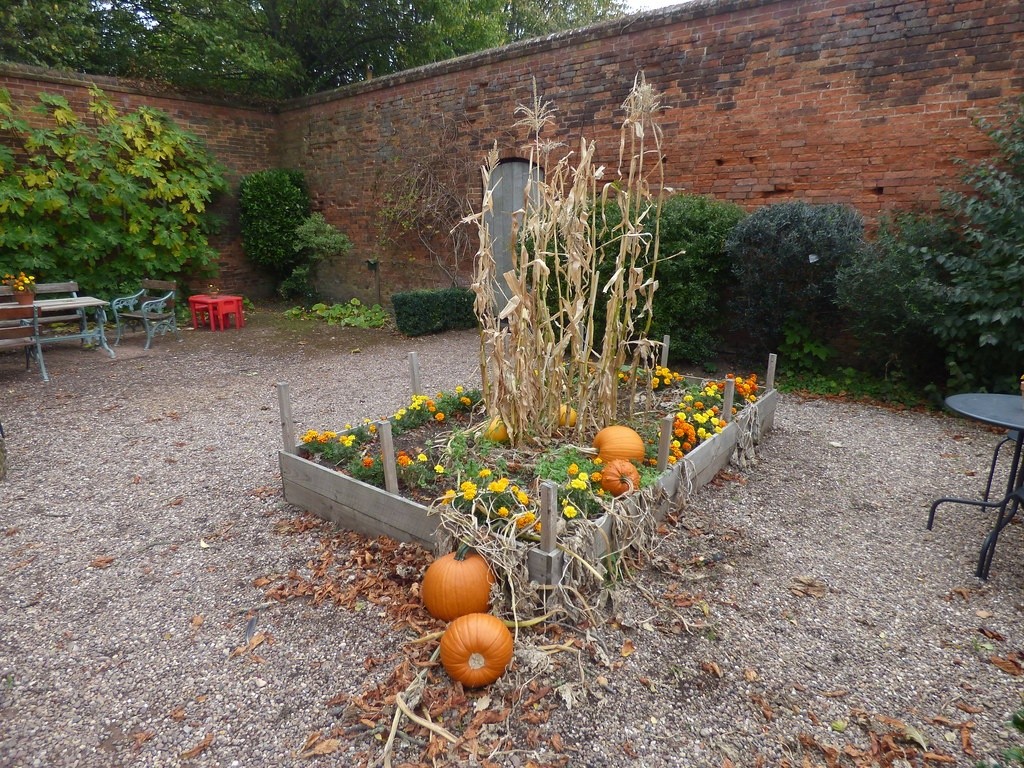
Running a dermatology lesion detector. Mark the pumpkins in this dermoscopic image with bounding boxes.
[423,539,496,622]
[441,613,514,688]
[559,405,577,426]
[576,426,645,464]
[601,460,639,498]
[482,415,508,442]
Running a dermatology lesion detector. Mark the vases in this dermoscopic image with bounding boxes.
[15,291,34,305]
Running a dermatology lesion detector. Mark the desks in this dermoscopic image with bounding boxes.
[188,294,243,331]
[0,297,116,359]
[928,392,1024,579]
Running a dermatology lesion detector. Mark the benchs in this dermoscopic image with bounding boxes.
[0,305,49,381]
[0,282,88,345]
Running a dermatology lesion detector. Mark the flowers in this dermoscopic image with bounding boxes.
[2,271,35,292]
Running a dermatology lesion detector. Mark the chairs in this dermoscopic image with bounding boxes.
[211,298,240,331]
[194,295,209,326]
[112,278,177,351]
